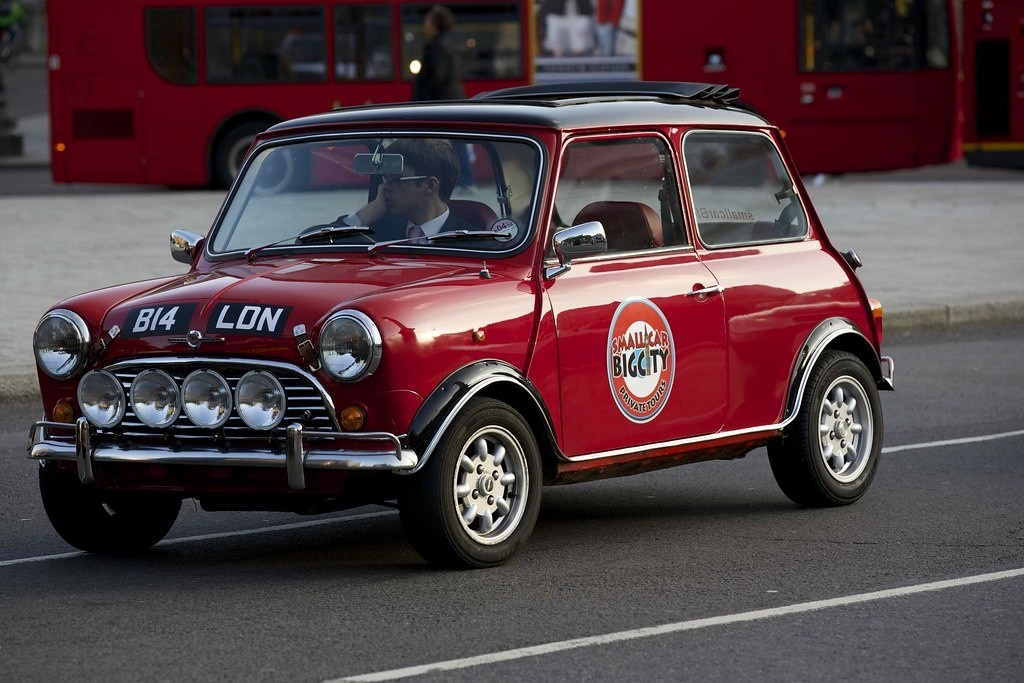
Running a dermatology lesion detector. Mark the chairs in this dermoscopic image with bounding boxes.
[572,200,663,250]
[450,199,498,229]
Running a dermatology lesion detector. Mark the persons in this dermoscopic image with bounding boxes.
[408,7,479,198]
[340,137,500,245]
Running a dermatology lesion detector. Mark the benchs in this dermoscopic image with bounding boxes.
[670,221,775,245]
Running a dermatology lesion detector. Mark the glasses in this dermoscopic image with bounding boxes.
[382,175,439,185]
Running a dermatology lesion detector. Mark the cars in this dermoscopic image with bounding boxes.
[24,80,896,570]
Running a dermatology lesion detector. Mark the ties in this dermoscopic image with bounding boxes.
[409,226,423,243]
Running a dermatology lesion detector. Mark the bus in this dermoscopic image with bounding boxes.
[47,0,963,196]
